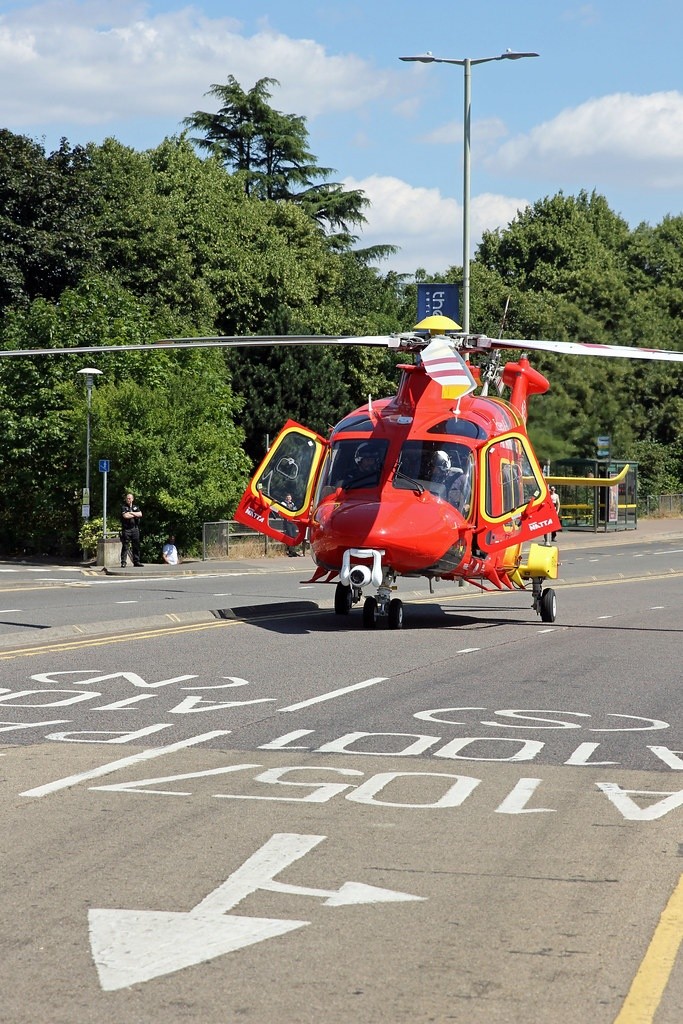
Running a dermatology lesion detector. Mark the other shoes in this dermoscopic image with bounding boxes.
[134,561,144,567]
[121,561,127,568]
[289,550,297,557]
[552,536,558,542]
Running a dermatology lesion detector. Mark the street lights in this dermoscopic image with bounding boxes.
[398,47,541,362]
[76,367,103,560]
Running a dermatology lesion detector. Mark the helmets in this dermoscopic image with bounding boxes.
[354,441,384,466]
[430,449,451,471]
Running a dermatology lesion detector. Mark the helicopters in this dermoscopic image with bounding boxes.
[0,307,683,631]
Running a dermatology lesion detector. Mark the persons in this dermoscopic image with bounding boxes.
[278,492,299,557]
[426,450,470,514]
[341,442,384,488]
[119,493,144,568]
[544,486,560,542]
